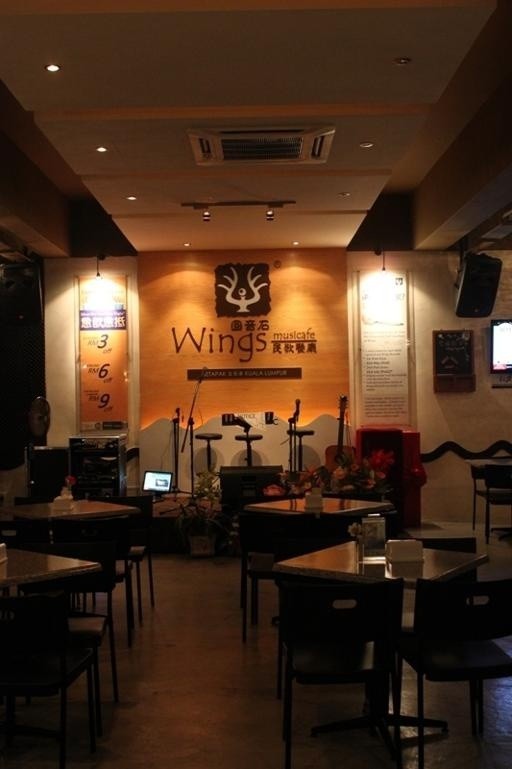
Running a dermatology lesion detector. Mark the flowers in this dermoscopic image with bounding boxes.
[262,447,396,508]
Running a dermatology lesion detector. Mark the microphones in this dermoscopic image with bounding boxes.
[196,364,208,386]
[295,399,301,420]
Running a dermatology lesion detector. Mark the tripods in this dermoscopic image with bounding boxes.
[159,382,221,515]
[153,408,200,502]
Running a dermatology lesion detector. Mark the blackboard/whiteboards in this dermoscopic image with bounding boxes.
[433,330,476,393]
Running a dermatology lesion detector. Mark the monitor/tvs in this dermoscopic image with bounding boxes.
[142,470,174,503]
[491,318,512,374]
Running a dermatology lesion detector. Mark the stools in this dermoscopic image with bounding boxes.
[194,429,314,499]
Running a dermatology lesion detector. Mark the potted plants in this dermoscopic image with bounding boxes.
[156,467,237,556]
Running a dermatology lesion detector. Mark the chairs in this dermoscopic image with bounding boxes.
[471,464,511,544]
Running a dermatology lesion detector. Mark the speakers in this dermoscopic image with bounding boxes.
[220,465,282,503]
[0,264,44,327]
[24,433,69,499]
[454,250,503,319]
[64,434,126,502]
